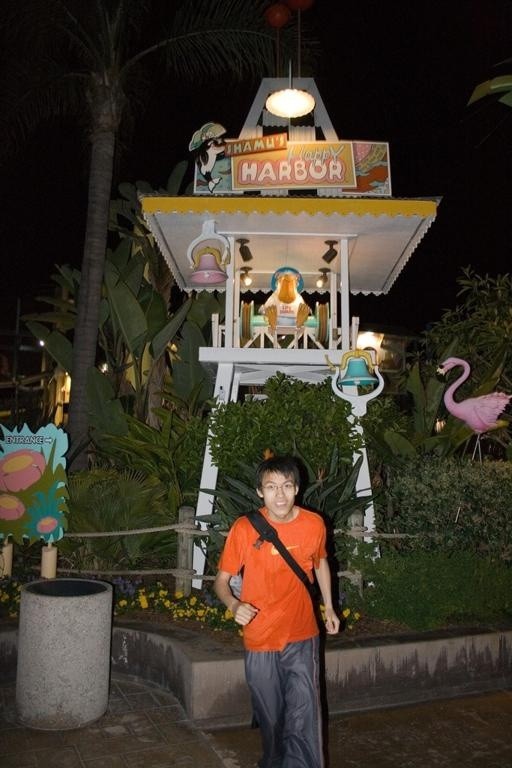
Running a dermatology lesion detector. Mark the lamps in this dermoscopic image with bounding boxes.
[236,238,253,261]
[322,239,339,263]
[240,266,254,287]
[265,54,316,120]
[316,268,331,288]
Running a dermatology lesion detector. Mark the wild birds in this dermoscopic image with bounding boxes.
[437,358,512,435]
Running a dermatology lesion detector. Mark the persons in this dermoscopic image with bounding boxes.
[213,456,343,767]
